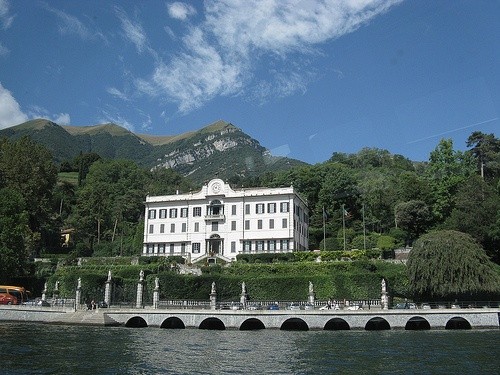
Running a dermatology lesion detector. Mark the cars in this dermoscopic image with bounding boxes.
[392,302,500,309]
[220,302,362,310]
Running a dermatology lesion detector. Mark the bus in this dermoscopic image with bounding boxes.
[0,285,30,305]
